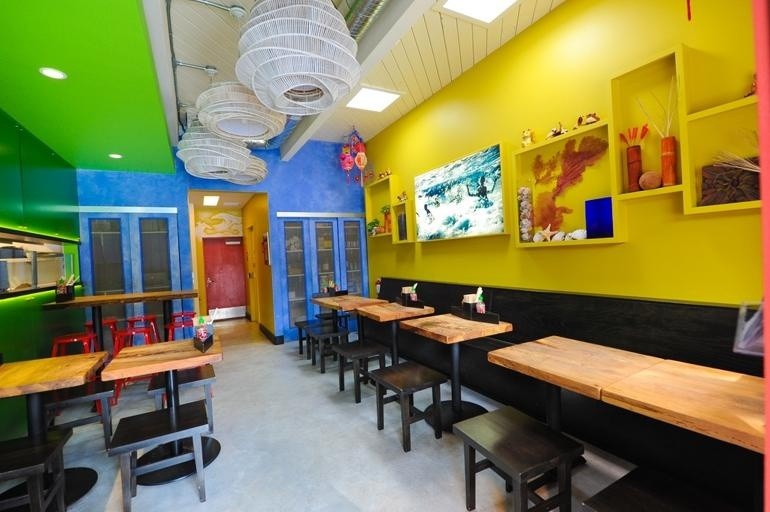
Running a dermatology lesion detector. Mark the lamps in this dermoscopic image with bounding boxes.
[176,100,252,179]
[217,156,269,186]
[186,0,361,117]
[173,57,287,143]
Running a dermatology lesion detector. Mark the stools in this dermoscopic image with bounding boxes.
[47,332,98,411]
[0,427,74,511]
[584,466,764,512]
[43,380,116,440]
[306,325,348,375]
[452,405,585,512]
[316,311,351,345]
[113,326,157,406]
[84,318,115,350]
[107,399,211,512]
[128,315,162,345]
[368,359,448,453]
[332,338,391,403]
[166,320,205,340]
[147,364,216,430]
[294,320,317,354]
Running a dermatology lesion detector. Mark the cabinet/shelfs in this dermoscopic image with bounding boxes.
[79,212,182,350]
[339,217,371,337]
[281,216,343,343]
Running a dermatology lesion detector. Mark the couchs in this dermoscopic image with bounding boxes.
[366,278,764,510]
